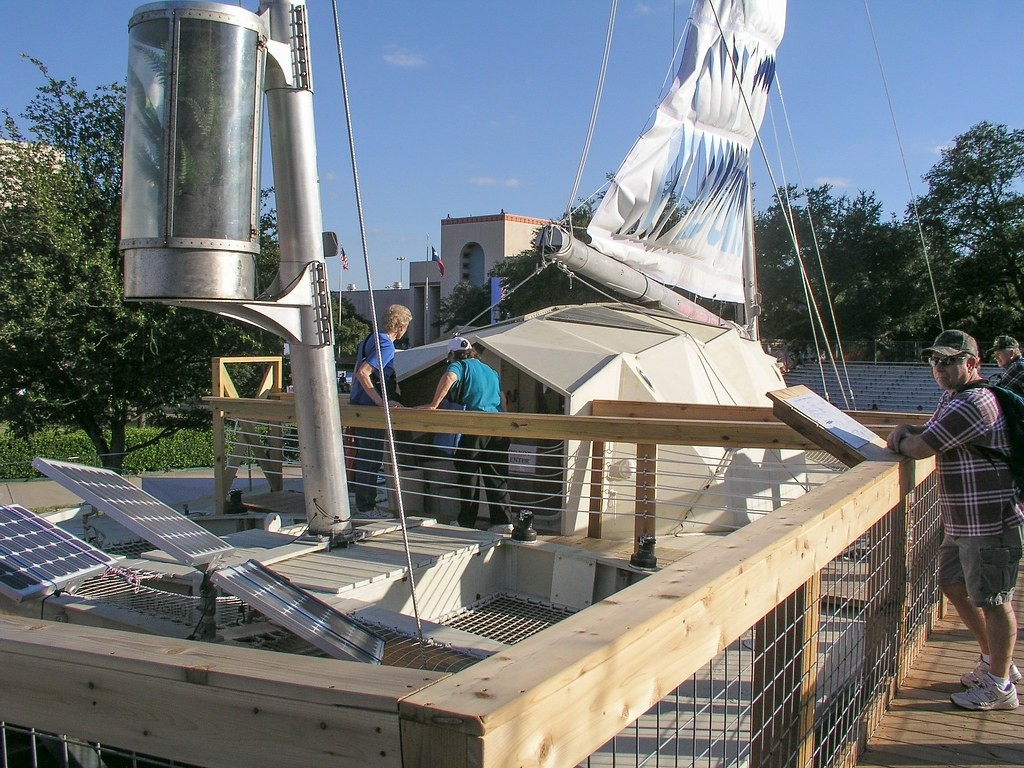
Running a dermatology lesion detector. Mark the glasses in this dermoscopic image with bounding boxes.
[928,353,969,366]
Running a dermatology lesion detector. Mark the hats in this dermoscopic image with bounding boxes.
[447,336,472,358]
[986,335,1019,354]
[921,330,978,357]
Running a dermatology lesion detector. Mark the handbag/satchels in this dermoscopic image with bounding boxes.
[432,358,466,454]
[370,372,403,403]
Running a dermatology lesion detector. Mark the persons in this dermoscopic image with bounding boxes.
[985,333,1024,398]
[884,328,1024,713]
[413,332,518,532]
[349,301,413,517]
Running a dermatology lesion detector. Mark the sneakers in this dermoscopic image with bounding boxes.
[950,672,1019,710]
[959,654,1022,688]
[450,520,460,527]
[375,493,387,502]
[487,524,515,534]
[356,507,395,518]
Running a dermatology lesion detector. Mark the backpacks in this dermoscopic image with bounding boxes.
[957,374,1024,499]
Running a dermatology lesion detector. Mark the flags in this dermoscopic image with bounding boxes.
[340,246,349,271]
[431,246,445,277]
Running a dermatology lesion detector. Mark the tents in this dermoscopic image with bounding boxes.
[385,299,817,540]
